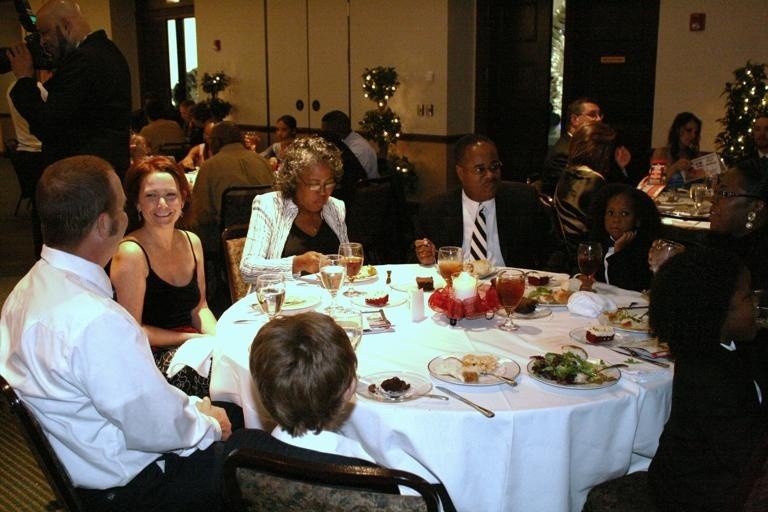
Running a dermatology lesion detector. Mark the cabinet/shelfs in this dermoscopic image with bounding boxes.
[264,0,350,137]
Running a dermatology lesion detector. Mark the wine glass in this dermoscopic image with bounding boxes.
[496,269,526,331]
[690,184,708,213]
[318,254,346,311]
[753,288,768,329]
[338,242,364,296]
[639,239,684,302]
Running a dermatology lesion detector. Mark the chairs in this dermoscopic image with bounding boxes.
[526,177,574,273]
[220,223,253,303]
[221,448,440,512]
[0,377,84,512]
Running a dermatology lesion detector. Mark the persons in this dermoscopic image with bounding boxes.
[109,156,218,400]
[239,133,354,294]
[539,97,768,309]
[413,135,554,270]
[5,0,132,185]
[129,82,381,228]
[1,155,244,512]
[250,311,379,465]
[649,254,768,512]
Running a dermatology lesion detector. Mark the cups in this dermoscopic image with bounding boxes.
[438,247,463,287]
[246,131,255,146]
[328,308,363,353]
[256,274,286,322]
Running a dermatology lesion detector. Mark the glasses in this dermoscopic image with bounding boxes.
[298,176,337,191]
[470,161,503,175]
[576,113,605,121]
[716,191,759,199]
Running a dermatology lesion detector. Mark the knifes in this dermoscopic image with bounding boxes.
[386,270,392,284]
[610,348,669,368]
[435,386,495,418]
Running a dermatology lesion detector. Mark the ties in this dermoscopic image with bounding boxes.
[468,203,487,263]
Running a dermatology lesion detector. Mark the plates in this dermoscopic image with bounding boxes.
[569,327,633,347]
[495,305,551,320]
[527,356,622,390]
[316,264,378,283]
[525,287,570,307]
[428,353,521,386]
[257,287,321,311]
[356,371,433,403]
[598,308,648,333]
